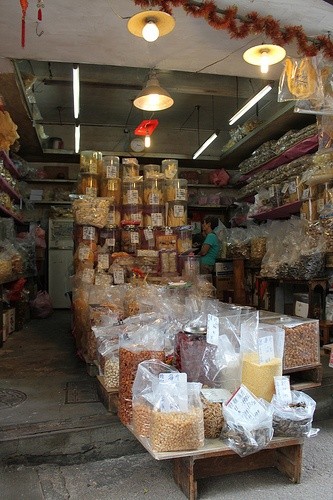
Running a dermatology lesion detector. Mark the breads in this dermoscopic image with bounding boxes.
[161,253,177,272]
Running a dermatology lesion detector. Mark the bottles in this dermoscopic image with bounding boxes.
[48,137,64,149]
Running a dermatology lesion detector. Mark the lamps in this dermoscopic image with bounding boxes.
[242,38,289,73]
[131,64,175,112]
[227,74,276,127]
[70,62,82,126]
[75,122,83,155]
[192,95,221,159]
[125,6,178,43]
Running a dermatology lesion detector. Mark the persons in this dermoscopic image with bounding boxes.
[198,215,220,274]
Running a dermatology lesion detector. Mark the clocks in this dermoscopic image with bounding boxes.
[127,136,147,153]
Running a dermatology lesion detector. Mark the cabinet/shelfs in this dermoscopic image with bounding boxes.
[178,73,333,311]
[0,65,79,316]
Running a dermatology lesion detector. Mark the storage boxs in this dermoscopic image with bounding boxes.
[252,310,321,370]
